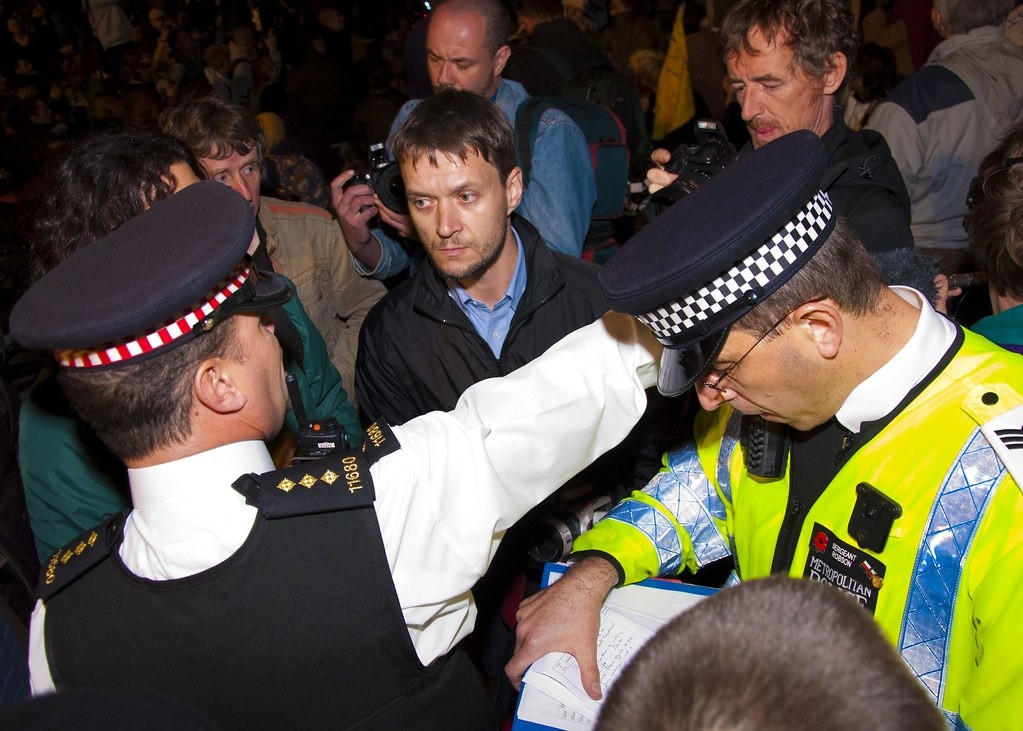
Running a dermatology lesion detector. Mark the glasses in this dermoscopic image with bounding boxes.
[695,294,828,394]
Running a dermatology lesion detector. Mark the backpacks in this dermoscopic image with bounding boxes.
[514,97,629,239]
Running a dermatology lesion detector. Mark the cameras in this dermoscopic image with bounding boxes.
[353,143,409,217]
[524,495,612,561]
[643,141,728,219]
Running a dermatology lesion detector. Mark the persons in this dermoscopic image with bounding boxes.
[352,87,674,724]
[0,0,1023,722]
[10,179,661,731]
[502,128,1023,731]
[644,1,910,269]
[592,571,951,730]
[18,127,365,567]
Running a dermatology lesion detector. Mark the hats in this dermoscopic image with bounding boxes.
[597,129,836,397]
[9,179,293,371]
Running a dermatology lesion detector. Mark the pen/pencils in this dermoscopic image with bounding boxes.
[947,272,988,288]
